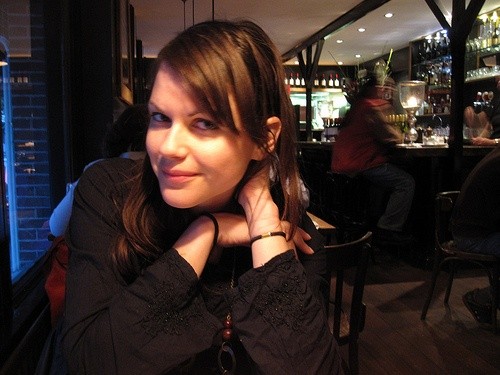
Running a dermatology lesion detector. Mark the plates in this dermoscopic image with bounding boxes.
[421,144,446,147]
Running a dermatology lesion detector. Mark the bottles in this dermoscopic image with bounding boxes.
[321,74,326,88]
[415,41,432,63]
[329,73,333,88]
[289,73,294,87]
[314,73,319,87]
[417,126,450,137]
[431,35,450,59]
[284,73,288,84]
[322,118,343,138]
[335,74,340,87]
[419,65,451,85]
[481,20,500,48]
[301,77,305,87]
[295,73,300,87]
[425,96,436,114]
[386,114,408,133]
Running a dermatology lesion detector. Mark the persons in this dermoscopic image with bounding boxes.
[49,104,149,237]
[330,74,415,236]
[463,76,500,145]
[450,145,500,329]
[53,20,348,374]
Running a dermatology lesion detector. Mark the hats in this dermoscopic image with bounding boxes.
[363,74,398,93]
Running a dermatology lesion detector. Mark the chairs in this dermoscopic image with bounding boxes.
[318,231,377,375]
[421,147,500,337]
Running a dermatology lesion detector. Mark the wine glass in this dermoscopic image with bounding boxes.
[409,134,418,146]
[476,92,493,102]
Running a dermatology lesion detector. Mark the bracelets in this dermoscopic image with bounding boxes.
[249,231,286,245]
[200,211,219,245]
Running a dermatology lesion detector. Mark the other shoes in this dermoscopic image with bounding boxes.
[373,227,417,246]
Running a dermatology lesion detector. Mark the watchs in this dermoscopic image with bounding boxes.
[494,138,499,145]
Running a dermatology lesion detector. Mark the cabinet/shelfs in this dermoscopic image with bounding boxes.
[406,7,500,137]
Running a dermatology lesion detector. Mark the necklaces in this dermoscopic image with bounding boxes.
[223,252,239,343]
